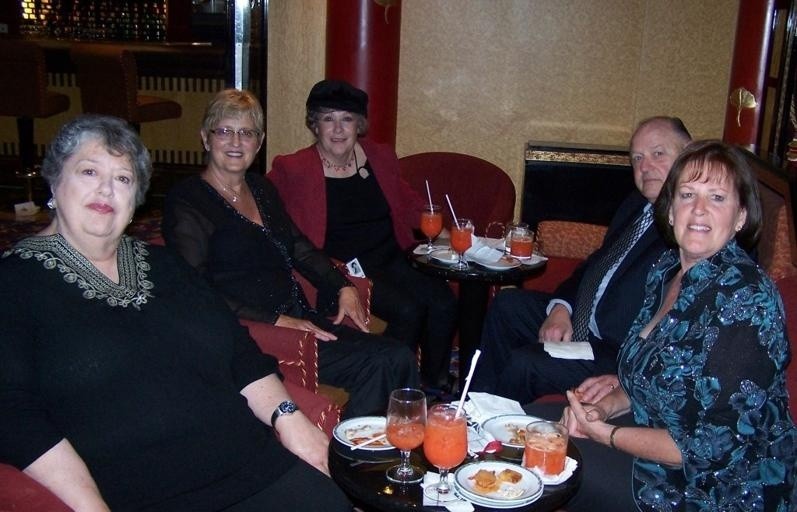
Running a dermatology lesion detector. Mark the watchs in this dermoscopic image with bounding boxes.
[266,398,301,433]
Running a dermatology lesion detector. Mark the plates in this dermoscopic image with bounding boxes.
[332,416,398,451]
[453,459,545,510]
[429,250,457,264]
[474,254,522,271]
[481,414,548,449]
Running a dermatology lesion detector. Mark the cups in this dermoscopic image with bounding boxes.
[525,420,570,475]
[507,222,535,259]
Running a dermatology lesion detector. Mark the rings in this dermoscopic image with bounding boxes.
[363,320,369,325]
[608,383,615,389]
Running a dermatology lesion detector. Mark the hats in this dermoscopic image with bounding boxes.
[306,80,368,119]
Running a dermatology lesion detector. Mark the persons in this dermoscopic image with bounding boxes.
[157,88,426,419]
[347,261,361,275]
[520,138,797,512]
[0,113,357,512]
[263,77,464,395]
[464,114,695,404]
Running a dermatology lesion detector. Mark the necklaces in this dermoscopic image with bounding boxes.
[316,142,360,171]
[215,175,249,204]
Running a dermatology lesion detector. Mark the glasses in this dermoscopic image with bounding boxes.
[211,127,260,140]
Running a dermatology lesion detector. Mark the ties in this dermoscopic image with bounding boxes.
[571,204,654,341]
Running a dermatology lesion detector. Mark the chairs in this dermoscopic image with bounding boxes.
[397,150,516,237]
[1,34,182,191]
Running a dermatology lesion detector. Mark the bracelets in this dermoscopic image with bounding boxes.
[611,422,621,448]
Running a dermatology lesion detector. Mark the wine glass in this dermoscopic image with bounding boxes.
[423,404,469,502]
[384,388,428,484]
[421,205,444,251]
[451,220,472,270]
